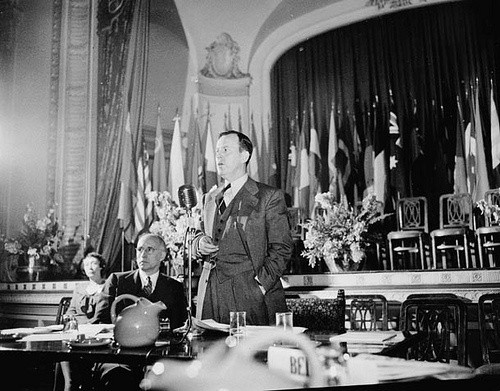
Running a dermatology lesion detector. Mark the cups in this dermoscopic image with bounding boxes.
[229,311,246,339]
[276,312,293,330]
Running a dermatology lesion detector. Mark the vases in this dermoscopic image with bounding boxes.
[18,252,56,282]
[323,255,363,271]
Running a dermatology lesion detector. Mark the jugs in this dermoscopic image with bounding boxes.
[110,294,167,347]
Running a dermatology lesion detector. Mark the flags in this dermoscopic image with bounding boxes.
[117,107,279,245]
[282,79,500,230]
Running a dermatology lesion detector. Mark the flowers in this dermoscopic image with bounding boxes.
[2,200,70,261]
[476,199,500,225]
[149,188,208,275]
[297,192,393,265]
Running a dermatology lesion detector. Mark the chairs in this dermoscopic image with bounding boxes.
[384,189,500,271]
[289,290,500,367]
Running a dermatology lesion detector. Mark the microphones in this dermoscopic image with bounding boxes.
[178,184,197,211]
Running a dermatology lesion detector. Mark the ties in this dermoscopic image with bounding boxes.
[143,276,152,298]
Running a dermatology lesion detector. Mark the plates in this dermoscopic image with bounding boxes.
[69,337,112,347]
[276,341,322,348]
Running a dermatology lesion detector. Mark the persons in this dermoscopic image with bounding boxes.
[54,252,107,391]
[187,130,295,326]
[90,234,187,391]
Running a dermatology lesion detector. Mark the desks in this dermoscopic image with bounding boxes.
[0,331,193,391]
[273,362,480,390]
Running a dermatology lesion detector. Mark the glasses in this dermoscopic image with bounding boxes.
[135,247,163,255]
